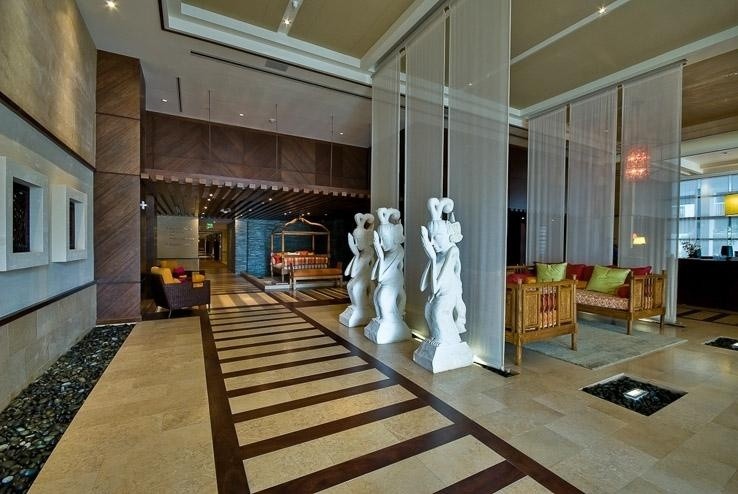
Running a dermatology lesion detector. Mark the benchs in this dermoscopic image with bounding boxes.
[506,262,666,364]
[289,262,343,290]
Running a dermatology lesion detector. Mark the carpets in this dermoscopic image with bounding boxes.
[508,317,686,370]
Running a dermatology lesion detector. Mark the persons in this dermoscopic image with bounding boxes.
[416,196,469,342]
[366,205,409,319]
[341,211,377,309]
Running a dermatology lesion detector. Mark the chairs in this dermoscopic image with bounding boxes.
[144,261,210,319]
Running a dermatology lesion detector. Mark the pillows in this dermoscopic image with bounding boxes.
[271,255,281,264]
[506,261,653,305]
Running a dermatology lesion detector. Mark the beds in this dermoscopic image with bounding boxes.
[270,251,328,283]
